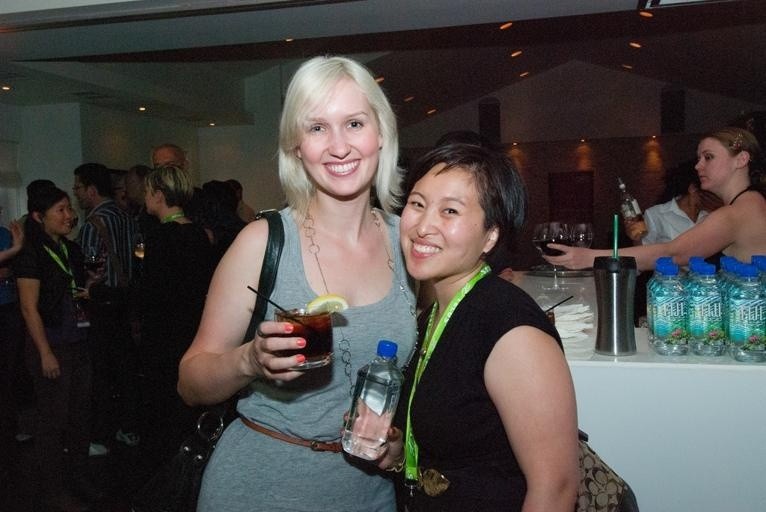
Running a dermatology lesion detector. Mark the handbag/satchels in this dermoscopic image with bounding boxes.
[573,429,639,511]
[128,207,285,511]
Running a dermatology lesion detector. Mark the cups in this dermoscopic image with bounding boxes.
[572,223,592,247]
[594,256,638,356]
[274,303,334,372]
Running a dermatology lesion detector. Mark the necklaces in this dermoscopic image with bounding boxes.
[300,207,418,400]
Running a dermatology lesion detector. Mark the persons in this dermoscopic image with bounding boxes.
[176,56,424,512]
[624,170,721,246]
[2,142,254,512]
[340,143,579,511]
[541,128,766,272]
[431,130,514,281]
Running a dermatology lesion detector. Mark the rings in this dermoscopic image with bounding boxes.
[572,265,576,269]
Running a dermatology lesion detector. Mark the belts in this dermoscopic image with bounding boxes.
[239,413,342,454]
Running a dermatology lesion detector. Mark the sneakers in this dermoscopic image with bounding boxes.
[114,429,139,448]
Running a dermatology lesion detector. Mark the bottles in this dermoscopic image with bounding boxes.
[646,255,766,363]
[616,177,649,239]
[341,339,405,462]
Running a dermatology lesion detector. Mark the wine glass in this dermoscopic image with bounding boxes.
[533,222,571,289]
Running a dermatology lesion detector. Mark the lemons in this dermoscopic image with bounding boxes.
[306,293,349,316]
